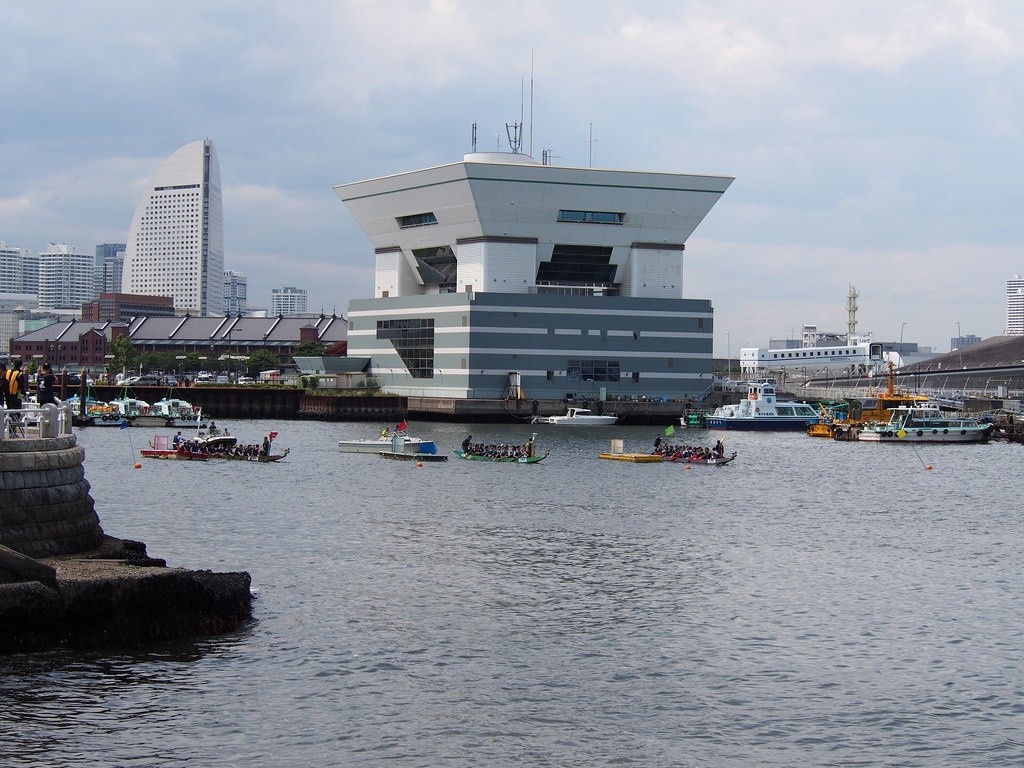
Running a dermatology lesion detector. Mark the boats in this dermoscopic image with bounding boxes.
[20,369,291,463]
[649,437,738,466]
[806,361,993,444]
[535,406,619,426]
[337,429,438,457]
[450,445,551,464]
[702,382,822,433]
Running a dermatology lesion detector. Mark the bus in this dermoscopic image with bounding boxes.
[757,377,778,390]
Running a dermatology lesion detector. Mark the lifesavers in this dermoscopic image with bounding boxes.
[187,416,191,420]
[686,403,691,408]
[193,416,197,420]
[102,416,107,421]
[917,430,923,436]
[181,416,186,420]
[597,400,604,412]
[837,428,842,436]
[713,403,718,408]
[933,429,937,434]
[882,433,886,437]
[114,415,119,420]
[109,415,114,420]
[584,401,588,407]
[887,431,893,437]
[756,407,760,412]
[961,430,967,435]
[895,430,908,437]
[532,400,539,411]
[132,416,135,421]
[943,429,948,434]
[200,417,203,420]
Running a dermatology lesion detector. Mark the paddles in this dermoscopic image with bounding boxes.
[483,451,527,462]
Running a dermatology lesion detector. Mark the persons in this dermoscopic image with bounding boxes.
[156,377,169,386]
[653,434,724,459]
[0,363,11,408]
[173,422,271,458]
[462,435,532,458]
[23,367,29,392]
[35,362,54,406]
[382,425,399,437]
[6,359,26,438]
[185,378,190,387]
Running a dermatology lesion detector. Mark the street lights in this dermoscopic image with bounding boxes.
[725,331,731,379]
[954,322,962,368]
[896,322,907,375]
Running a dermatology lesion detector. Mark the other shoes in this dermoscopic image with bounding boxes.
[10,433,19,438]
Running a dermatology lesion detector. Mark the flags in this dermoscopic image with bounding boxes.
[121,420,127,429]
[400,421,408,431]
[665,425,675,435]
[897,430,906,438]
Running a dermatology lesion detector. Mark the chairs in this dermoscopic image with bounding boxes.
[6,409,27,439]
